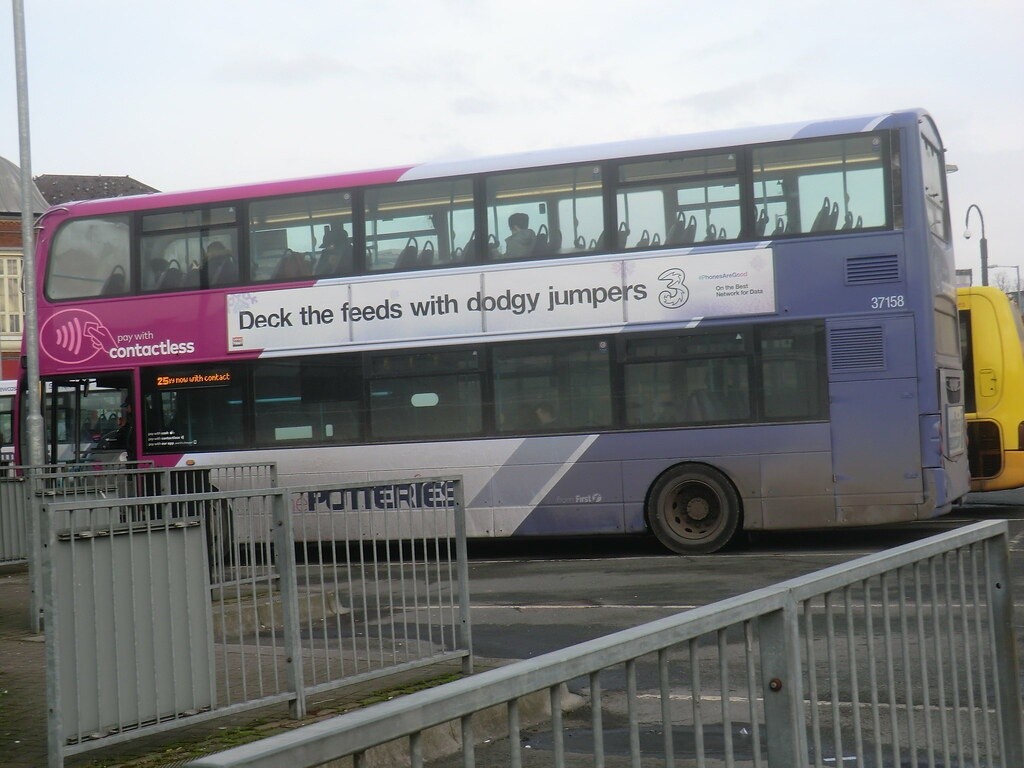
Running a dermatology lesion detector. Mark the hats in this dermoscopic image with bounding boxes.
[120,396,132,407]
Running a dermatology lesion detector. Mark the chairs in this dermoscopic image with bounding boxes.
[574,230,604,250]
[270,245,372,280]
[736,205,785,237]
[533,224,562,254]
[98,254,258,297]
[393,231,499,270]
[635,230,649,248]
[651,233,661,246]
[618,221,628,249]
[663,210,727,244]
[810,197,863,232]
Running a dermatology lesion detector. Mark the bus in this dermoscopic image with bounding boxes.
[956,285,1024,493]
[0,380,131,479]
[13,110,971,556]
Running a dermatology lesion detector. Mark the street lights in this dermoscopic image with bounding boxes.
[963,205,988,285]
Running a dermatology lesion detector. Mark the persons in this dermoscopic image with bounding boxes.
[492,213,535,259]
[191,241,231,288]
[535,401,559,428]
[116,395,149,463]
[327,231,352,271]
